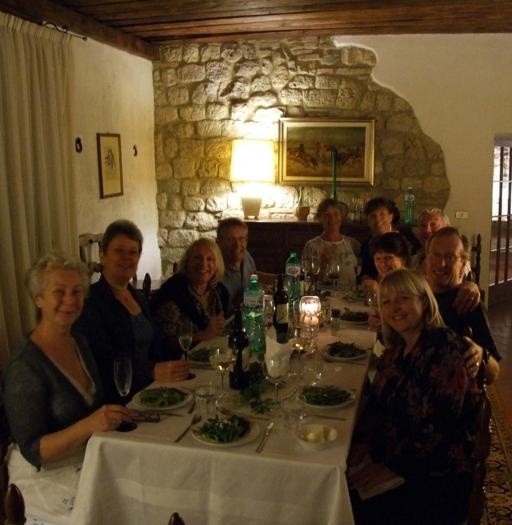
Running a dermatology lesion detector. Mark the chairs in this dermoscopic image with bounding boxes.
[78,232,105,275]
[470,233,485,307]
[0,442,25,525]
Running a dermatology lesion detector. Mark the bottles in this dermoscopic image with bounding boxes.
[293,186,310,221]
[348,195,370,223]
[403,186,416,224]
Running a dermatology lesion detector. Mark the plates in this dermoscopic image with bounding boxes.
[131,317,377,448]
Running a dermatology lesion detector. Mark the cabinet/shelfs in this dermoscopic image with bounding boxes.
[246,223,422,279]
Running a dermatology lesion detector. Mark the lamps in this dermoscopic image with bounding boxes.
[297,294,322,357]
[229,138,274,219]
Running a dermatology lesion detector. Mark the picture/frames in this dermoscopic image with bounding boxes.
[277,118,377,186]
[98,133,124,198]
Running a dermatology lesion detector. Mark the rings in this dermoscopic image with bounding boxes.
[175,378,178,382]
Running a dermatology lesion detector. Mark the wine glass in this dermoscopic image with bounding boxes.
[114,256,378,433]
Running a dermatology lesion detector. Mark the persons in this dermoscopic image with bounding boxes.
[302,196,502,384]
[344,270,493,525]
[149,238,240,343]
[215,218,256,304]
[71,219,191,406]
[2,250,133,525]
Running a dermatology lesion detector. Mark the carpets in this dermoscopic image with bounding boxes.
[481,381,511,525]
